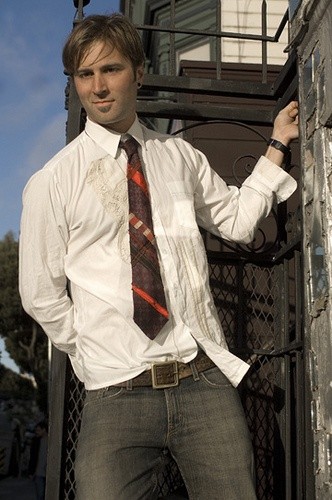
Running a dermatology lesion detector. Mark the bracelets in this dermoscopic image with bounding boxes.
[266,138,289,154]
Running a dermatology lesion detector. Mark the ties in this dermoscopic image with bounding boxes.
[119,137,170,341]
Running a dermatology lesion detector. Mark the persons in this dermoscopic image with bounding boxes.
[19,12,299,500]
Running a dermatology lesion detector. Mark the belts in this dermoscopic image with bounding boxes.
[110,349,215,390]
